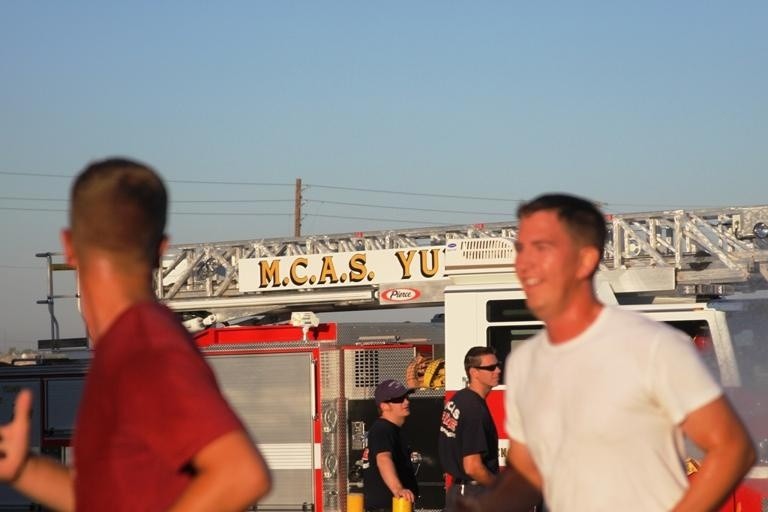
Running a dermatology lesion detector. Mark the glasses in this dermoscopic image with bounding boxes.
[473,360,502,372]
[386,393,410,403]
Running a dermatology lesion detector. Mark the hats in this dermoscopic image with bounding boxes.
[375,380,416,405]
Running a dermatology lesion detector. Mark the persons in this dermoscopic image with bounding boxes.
[360,376,419,512]
[505,194,758,510]
[434,347,503,511]
[1,155,274,511]
